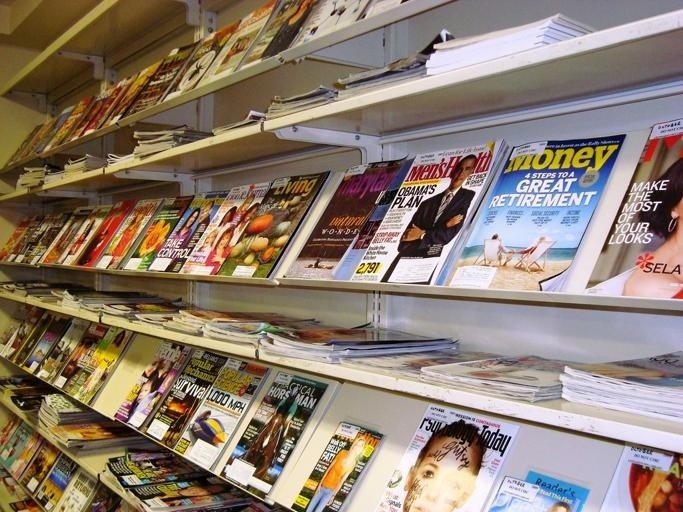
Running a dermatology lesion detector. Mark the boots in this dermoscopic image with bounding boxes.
[275,434,286,453]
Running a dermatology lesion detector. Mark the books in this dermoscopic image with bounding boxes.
[1,1,683,511]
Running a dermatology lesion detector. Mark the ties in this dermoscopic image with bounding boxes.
[434,193,453,224]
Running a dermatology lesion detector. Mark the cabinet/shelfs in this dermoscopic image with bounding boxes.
[0,0,683,512]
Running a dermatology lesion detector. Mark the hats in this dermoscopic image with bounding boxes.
[288,387,299,398]
